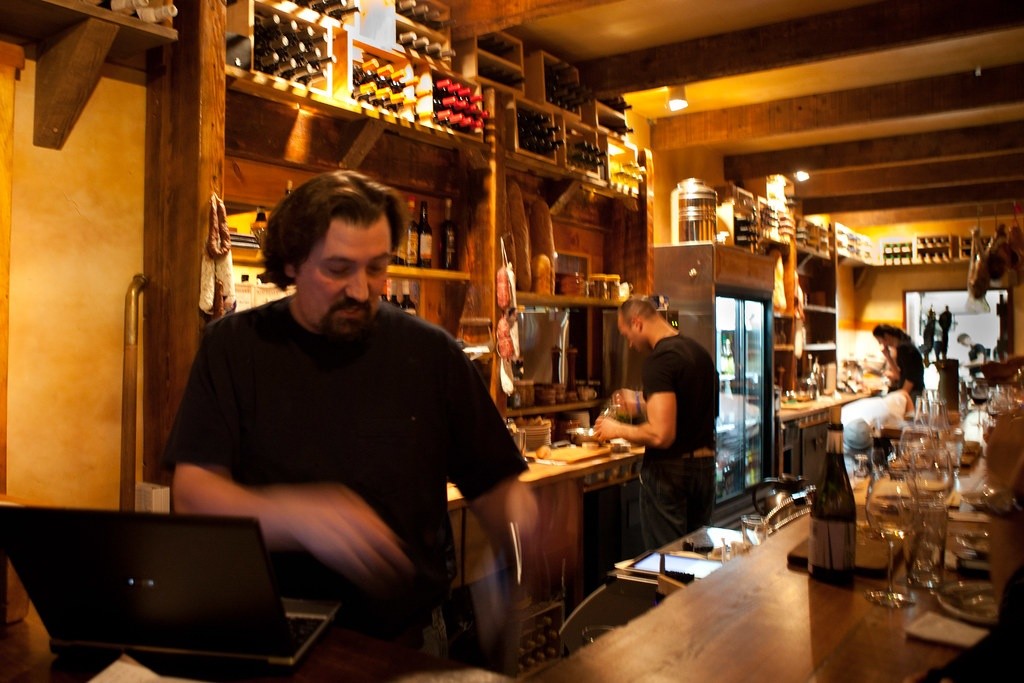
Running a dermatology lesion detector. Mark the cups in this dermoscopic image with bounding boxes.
[904,500,947,589]
[820,361,836,396]
[984,384,1019,419]
[958,467,1016,518]
[738,514,768,550]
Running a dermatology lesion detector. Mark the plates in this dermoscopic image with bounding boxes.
[516,412,591,450]
[938,580,997,624]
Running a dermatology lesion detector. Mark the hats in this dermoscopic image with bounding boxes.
[843,419,873,450]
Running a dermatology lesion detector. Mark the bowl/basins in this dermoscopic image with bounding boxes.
[566,428,602,444]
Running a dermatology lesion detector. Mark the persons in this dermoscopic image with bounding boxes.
[957,333,986,413]
[873,323,926,403]
[158,169,541,653]
[919,304,953,367]
[592,294,719,552]
[839,389,914,489]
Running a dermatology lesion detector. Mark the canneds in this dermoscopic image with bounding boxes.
[511,380,536,410]
[588,273,621,300]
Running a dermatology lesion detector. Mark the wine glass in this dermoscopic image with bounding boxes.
[864,423,956,608]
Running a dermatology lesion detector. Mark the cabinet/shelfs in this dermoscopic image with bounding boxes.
[144,0,1000,683]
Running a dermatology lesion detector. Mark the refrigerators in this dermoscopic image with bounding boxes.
[654,242,779,531]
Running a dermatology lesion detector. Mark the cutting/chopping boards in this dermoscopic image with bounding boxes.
[523,444,613,462]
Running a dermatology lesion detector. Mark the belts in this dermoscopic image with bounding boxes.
[681,447,717,460]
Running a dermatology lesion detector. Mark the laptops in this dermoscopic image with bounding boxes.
[0,505,342,674]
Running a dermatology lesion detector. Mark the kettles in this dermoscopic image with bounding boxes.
[753,472,812,527]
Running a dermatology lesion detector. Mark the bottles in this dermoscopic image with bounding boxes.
[379,278,416,318]
[252,207,268,236]
[515,380,565,405]
[733,208,810,245]
[911,389,948,423]
[283,179,296,197]
[557,272,622,301]
[808,406,857,589]
[390,194,458,269]
[101,1,645,184]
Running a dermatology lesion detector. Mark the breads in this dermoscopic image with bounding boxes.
[506,177,555,295]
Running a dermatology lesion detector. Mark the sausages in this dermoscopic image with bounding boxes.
[496,265,516,360]
[211,192,231,320]
